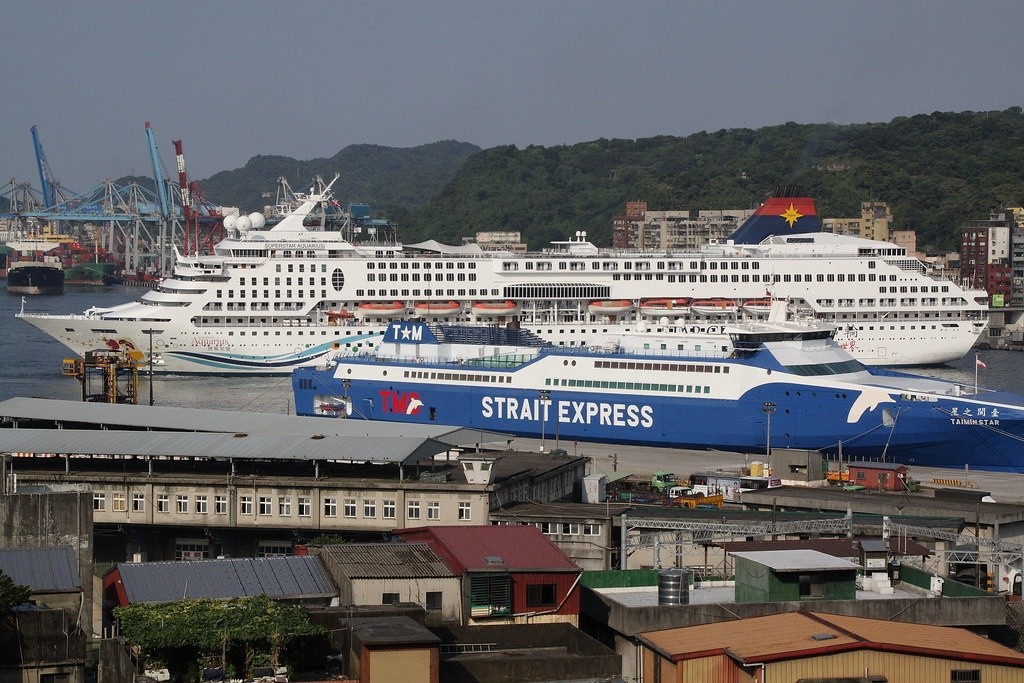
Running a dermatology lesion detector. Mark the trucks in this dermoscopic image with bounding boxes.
[668,486,693,499]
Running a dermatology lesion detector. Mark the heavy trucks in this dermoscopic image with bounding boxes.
[606,471,678,493]
[670,492,724,513]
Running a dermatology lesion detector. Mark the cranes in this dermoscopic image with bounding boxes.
[1,120,407,287]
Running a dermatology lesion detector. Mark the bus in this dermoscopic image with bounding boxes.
[688,471,782,504]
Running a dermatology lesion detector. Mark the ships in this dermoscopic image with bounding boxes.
[292,309,1024,472]
[12,170,991,378]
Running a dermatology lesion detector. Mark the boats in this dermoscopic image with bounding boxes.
[413,300,462,318]
[638,297,690,315]
[355,299,405,319]
[585,299,631,314]
[470,299,518,316]
[691,298,738,315]
[740,298,789,316]
[6,221,65,297]
